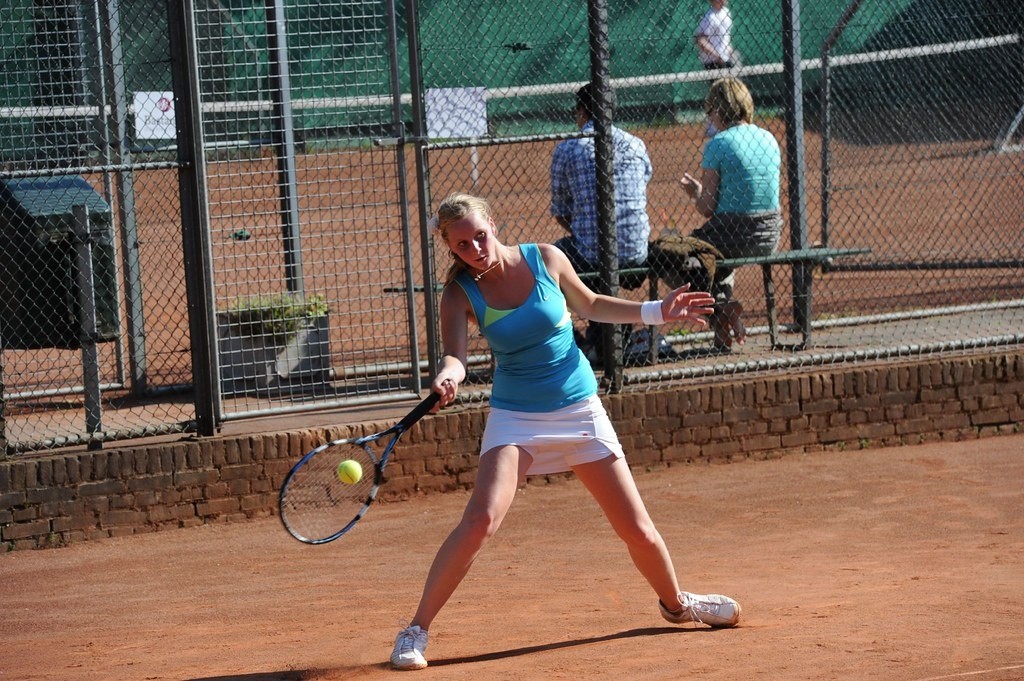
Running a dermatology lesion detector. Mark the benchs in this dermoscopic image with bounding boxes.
[384,245,870,365]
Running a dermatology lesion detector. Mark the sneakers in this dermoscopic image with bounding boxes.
[390,625,431,670]
[659,591,740,627]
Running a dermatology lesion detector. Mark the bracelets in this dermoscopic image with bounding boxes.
[640,300,668,326]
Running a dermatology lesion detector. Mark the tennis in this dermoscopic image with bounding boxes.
[335,460,363,486]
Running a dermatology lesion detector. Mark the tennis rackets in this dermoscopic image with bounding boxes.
[278,377,459,544]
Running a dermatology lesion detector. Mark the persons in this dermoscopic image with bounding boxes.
[548,84,656,362]
[694,1,741,135]
[389,191,742,670]
[650,77,784,358]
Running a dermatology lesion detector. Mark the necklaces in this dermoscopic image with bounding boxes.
[475,246,510,283]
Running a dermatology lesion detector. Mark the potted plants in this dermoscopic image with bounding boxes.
[213,289,331,390]
